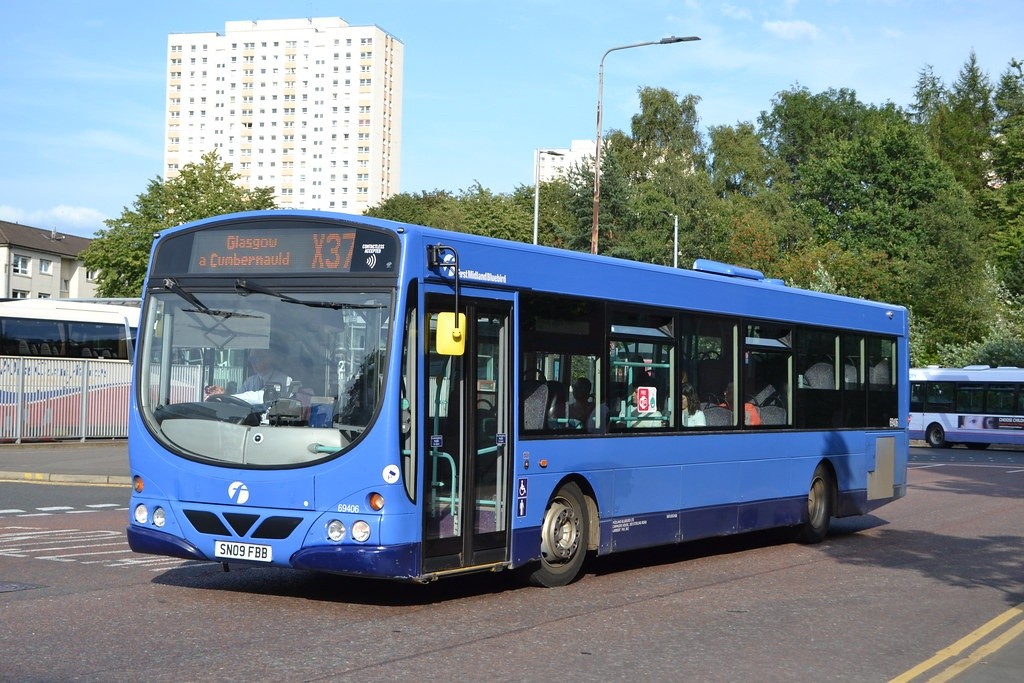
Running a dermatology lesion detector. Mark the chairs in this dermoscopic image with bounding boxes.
[41,343,52,355]
[30,344,38,354]
[81,347,92,356]
[51,346,59,355]
[113,352,118,357]
[19,340,31,354]
[798,351,892,389]
[101,350,111,357]
[93,351,98,356]
[524,350,788,430]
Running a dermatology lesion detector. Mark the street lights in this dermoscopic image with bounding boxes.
[533,148,562,243]
[591,35,699,253]
[658,210,679,266]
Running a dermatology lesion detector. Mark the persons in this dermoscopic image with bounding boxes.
[670,383,706,426]
[616,354,662,427]
[204,349,294,424]
[569,377,596,420]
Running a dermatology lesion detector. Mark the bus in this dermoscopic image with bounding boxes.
[911,364,1024,450]
[125,207,910,587]
[0,298,246,442]
